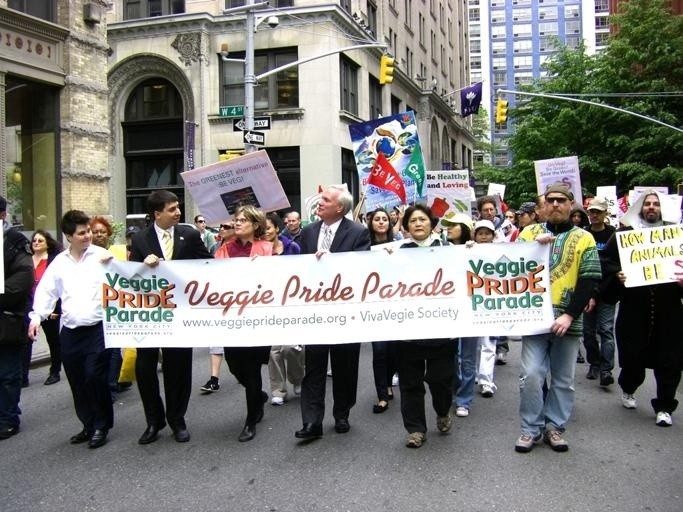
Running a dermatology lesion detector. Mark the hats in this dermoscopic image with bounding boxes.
[439,214,473,231]
[585,197,609,211]
[124,225,140,238]
[514,201,537,215]
[474,219,495,231]
[543,183,575,201]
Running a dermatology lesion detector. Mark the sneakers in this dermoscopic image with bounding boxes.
[543,430,571,452]
[654,411,672,427]
[270,396,284,406]
[454,406,469,417]
[620,392,638,409]
[480,385,494,397]
[292,383,301,396]
[495,352,506,365]
[435,410,452,433]
[599,370,614,387]
[405,431,427,448]
[198,380,221,392]
[585,367,599,379]
[513,428,542,454]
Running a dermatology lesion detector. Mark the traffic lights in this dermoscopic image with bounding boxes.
[379,55,394,86]
[495,97,509,124]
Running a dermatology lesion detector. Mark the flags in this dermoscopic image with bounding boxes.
[460,84,481,117]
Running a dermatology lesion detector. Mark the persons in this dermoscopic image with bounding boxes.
[1,183,682,454]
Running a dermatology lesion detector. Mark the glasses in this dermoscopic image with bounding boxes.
[545,196,571,203]
[219,223,233,230]
[231,217,251,224]
[195,220,205,223]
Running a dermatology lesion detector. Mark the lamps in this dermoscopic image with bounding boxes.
[12,124,21,184]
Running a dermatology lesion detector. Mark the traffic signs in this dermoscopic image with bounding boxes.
[217,106,244,118]
[231,116,272,132]
[242,130,264,145]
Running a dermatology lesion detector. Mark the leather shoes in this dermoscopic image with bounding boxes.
[293,422,323,440]
[238,407,262,444]
[136,421,167,444]
[371,401,388,414]
[255,391,268,423]
[69,426,92,444]
[43,375,60,385]
[333,415,350,434]
[88,428,106,447]
[387,385,394,401]
[0,420,20,440]
[174,429,190,441]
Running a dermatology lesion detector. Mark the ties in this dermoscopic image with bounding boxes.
[320,228,332,252]
[161,230,173,261]
